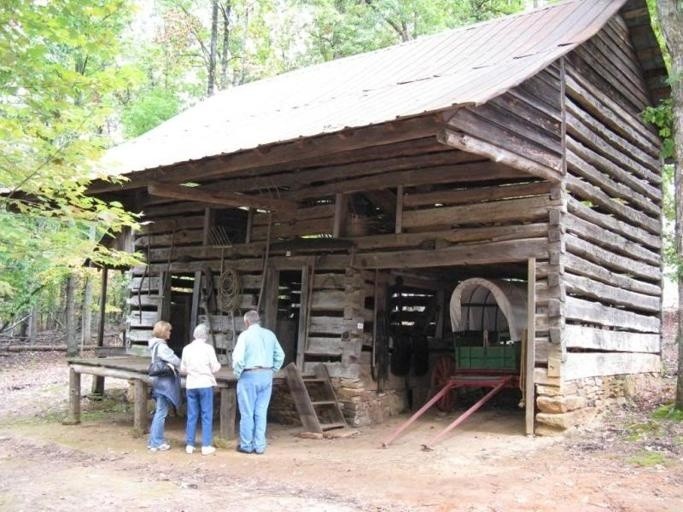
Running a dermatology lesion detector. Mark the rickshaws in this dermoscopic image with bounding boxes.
[381,277,528,450]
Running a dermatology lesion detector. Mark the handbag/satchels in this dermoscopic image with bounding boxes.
[147,342,179,378]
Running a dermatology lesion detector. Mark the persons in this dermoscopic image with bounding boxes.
[146,320,182,452]
[177,322,222,456]
[230,309,286,454]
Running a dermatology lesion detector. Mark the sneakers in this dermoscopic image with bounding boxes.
[235,442,265,454]
[146,440,171,453]
[200,444,217,456]
[185,444,197,454]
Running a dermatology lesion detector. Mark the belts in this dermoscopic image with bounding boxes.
[244,366,272,373]
